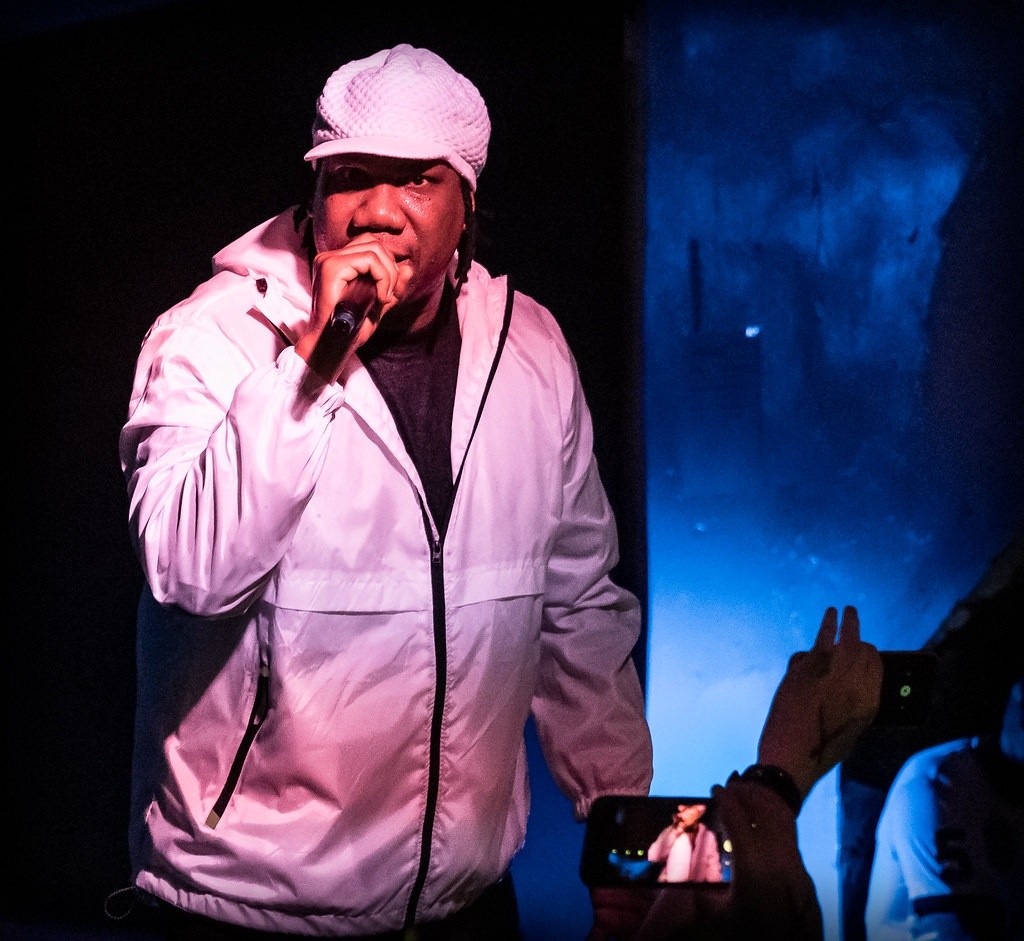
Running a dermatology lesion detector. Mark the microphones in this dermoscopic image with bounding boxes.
[329,271,381,337]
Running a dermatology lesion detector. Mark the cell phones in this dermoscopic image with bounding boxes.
[863,651,946,735]
[583,794,739,891]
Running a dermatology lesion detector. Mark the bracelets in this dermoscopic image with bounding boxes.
[742,764,802,820]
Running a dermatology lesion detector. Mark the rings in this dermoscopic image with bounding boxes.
[742,823,763,834]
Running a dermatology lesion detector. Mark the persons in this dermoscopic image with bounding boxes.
[648,804,722,882]
[119,42,654,941]
[584,606,1024,941]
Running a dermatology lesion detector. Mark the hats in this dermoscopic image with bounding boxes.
[304,43,491,193]
